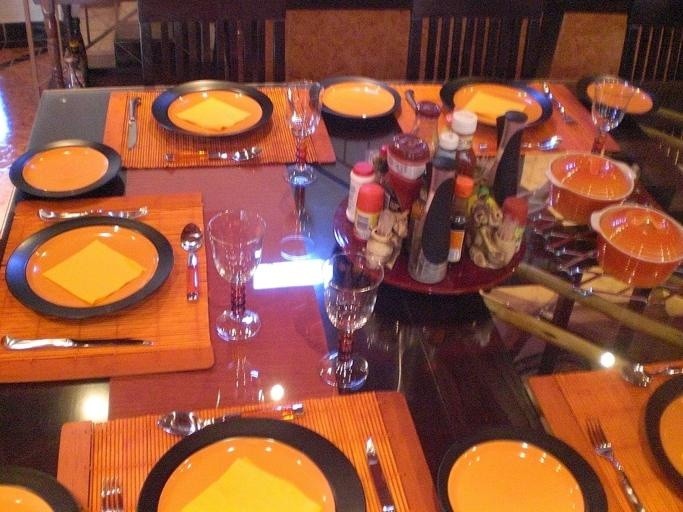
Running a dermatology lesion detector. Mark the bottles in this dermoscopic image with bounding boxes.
[62,16,89,89]
[345,100,529,283]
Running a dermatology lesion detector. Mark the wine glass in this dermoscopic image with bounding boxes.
[206,210,266,342]
[276,183,318,260]
[284,81,325,186]
[318,247,387,388]
[580,76,638,156]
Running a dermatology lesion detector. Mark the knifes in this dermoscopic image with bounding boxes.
[0,333,152,351]
[365,436,396,512]
[127,95,139,148]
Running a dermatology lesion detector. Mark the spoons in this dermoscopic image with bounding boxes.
[158,402,302,436]
[619,362,682,389]
[179,220,203,301]
[480,134,562,151]
[167,146,260,165]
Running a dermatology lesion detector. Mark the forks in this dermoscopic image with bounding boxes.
[100,476,124,509]
[539,76,575,125]
[38,203,147,221]
[583,413,646,511]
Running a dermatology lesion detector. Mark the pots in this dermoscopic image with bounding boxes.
[584,204,682,290]
[541,149,641,222]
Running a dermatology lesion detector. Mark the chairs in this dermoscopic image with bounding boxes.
[137,0,285,85]
[406,0,564,81]
[618,1,683,82]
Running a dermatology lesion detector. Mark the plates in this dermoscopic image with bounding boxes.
[306,75,402,121]
[150,79,272,138]
[439,79,553,135]
[0,461,84,512]
[10,137,122,200]
[333,195,526,300]
[644,377,683,495]
[577,72,658,119]
[436,426,610,512]
[137,418,365,509]
[5,215,172,319]
